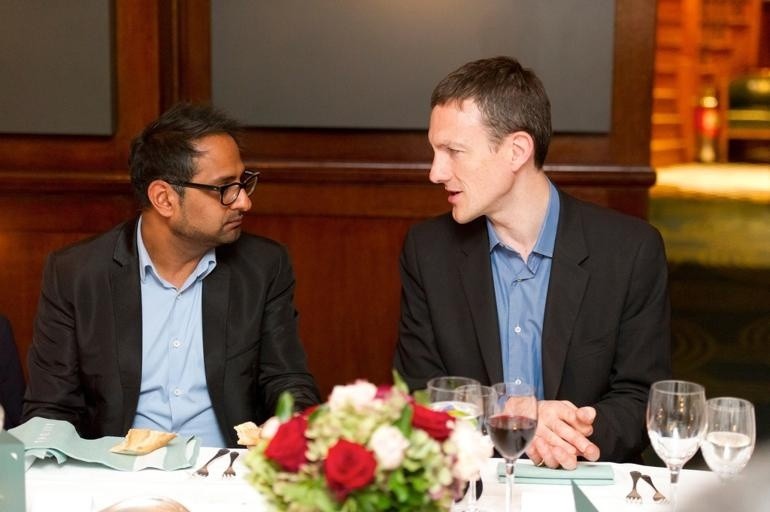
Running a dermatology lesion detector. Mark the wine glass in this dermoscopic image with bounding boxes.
[455,382,538,512]
[646,380,709,511]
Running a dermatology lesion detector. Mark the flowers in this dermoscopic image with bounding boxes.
[233,375,498,512]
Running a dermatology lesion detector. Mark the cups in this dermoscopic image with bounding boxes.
[700,396,757,484]
[427,376,484,408]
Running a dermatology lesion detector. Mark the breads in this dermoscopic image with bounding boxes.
[111,428,178,456]
[230,420,259,448]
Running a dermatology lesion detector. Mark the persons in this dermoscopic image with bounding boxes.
[386,55,676,475]
[17,98,324,452]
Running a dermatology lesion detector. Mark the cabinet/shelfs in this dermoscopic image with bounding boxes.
[647,0,770,186]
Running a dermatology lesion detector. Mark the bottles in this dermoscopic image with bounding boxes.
[694,72,722,162]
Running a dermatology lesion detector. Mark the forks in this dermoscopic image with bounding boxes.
[188,443,238,479]
[623,470,674,507]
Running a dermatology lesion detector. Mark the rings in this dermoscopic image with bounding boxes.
[536,459,546,467]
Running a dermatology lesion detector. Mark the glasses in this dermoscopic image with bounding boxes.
[163,171,261,207]
